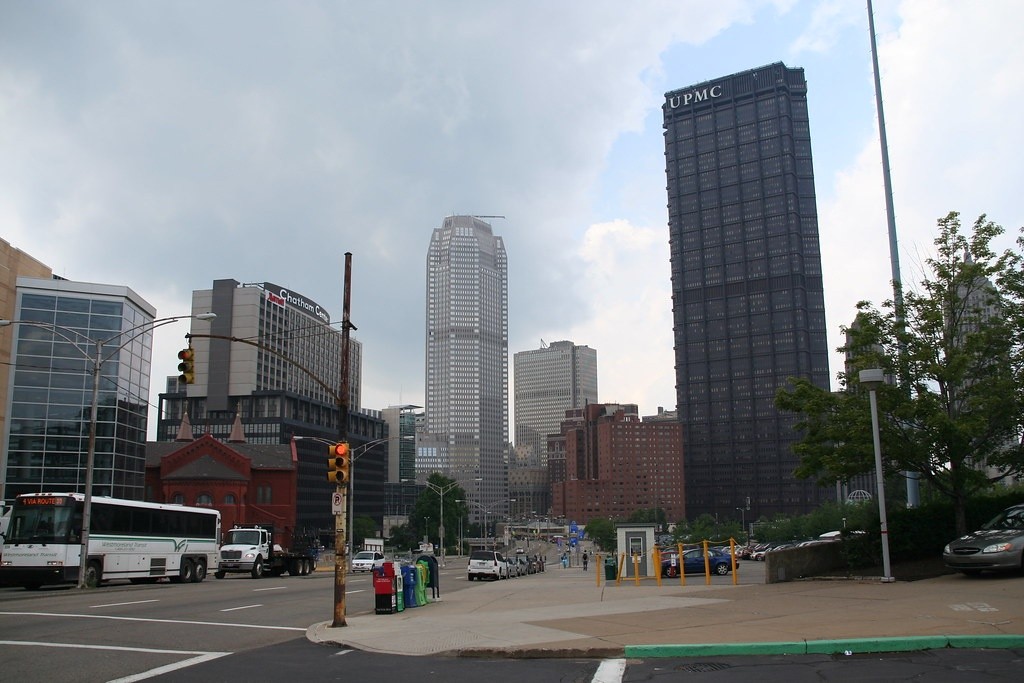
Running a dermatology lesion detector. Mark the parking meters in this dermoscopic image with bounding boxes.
[371,560,431,614]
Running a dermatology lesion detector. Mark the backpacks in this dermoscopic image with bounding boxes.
[583,554,587,560]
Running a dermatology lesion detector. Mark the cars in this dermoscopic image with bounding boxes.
[655,542,714,561]
[662,548,739,578]
[942,505,1024,578]
[715,541,832,561]
[503,555,546,578]
[351,550,390,572]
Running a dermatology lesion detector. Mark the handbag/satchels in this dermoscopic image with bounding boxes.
[561,556,564,560]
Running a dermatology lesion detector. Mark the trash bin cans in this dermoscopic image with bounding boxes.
[604,557,617,580]
[372,555,439,614]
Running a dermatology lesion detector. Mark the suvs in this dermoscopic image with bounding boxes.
[467,551,510,581]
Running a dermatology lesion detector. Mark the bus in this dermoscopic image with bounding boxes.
[0,492,222,590]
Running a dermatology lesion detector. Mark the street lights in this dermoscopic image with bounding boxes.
[736,508,745,531]
[424,517,430,535]
[455,499,516,550]
[859,368,895,582]
[293,435,414,574]
[0,312,218,591]
[401,479,482,561]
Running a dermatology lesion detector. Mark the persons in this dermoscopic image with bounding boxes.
[582,550,588,571]
[562,553,568,569]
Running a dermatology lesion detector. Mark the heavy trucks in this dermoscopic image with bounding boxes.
[215,526,317,579]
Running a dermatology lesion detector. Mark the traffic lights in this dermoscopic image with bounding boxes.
[327,446,336,482]
[335,443,349,484]
[178,348,196,384]
